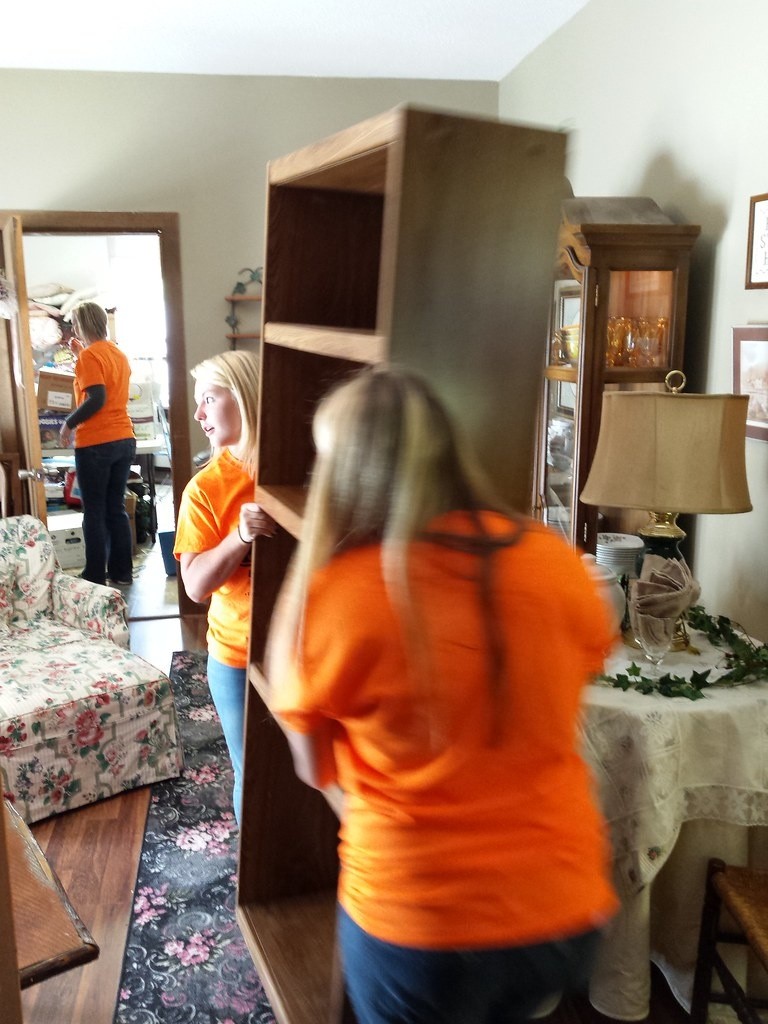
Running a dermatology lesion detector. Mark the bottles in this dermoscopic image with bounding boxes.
[606,313,668,369]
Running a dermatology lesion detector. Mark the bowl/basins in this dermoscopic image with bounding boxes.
[560,323,582,365]
[547,416,574,468]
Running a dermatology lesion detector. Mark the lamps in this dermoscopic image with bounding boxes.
[583,373,754,652]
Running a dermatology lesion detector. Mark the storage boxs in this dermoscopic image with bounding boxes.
[37,366,158,574]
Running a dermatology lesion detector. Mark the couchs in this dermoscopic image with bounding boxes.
[0,514,185,831]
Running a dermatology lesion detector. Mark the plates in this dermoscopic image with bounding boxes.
[542,505,645,577]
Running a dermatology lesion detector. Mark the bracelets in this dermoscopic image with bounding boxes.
[238,525,252,544]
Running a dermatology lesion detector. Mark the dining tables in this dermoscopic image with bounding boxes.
[569,611,768,1024]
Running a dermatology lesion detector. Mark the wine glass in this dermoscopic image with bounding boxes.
[628,607,675,678]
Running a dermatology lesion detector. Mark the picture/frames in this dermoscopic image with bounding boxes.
[733,323,768,443]
[745,193,768,290]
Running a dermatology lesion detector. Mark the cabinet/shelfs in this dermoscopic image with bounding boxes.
[231,104,573,1024]
[223,290,264,352]
[535,195,706,553]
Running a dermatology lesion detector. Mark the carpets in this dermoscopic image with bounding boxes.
[111,649,279,1024]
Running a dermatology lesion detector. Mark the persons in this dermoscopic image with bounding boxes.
[59,301,137,586]
[262,365,622,1024]
[173,348,277,832]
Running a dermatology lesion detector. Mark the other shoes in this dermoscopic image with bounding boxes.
[105,571,133,585]
[74,574,83,578]
